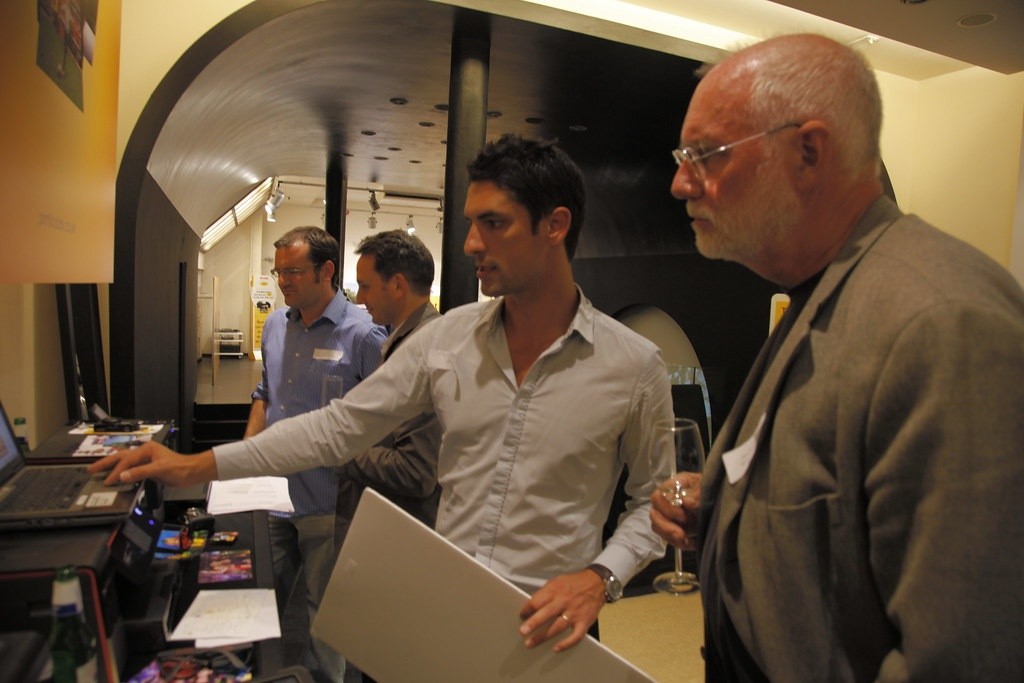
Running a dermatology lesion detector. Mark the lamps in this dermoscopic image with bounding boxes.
[407,215,416,233]
[265,190,284,222]
[367,212,377,228]
[437,217,443,233]
[369,191,380,210]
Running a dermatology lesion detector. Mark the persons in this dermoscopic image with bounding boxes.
[326,229,444,683]
[240,224,388,683]
[86,132,678,683]
[651,32,1024,683]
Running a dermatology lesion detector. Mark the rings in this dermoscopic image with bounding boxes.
[560,614,571,627]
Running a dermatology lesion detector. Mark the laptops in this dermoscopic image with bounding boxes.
[0,400,147,524]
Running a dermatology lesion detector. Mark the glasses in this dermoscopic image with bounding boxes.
[270,265,320,275]
[672,123,805,178]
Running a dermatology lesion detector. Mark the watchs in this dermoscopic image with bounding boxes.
[585,563,623,604]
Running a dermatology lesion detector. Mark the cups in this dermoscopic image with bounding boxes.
[321,377,343,407]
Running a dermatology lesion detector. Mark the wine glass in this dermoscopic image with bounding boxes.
[646,418,705,595]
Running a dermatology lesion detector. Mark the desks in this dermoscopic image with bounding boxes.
[213,331,244,359]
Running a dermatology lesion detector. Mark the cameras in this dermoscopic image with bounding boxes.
[179,505,217,530]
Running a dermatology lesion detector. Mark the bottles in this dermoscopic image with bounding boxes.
[49,565,97,682]
